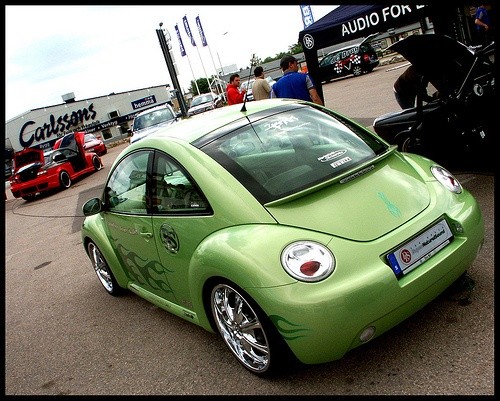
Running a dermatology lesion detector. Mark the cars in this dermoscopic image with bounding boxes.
[80,97,484,380]
[187,92,222,117]
[5,147,13,178]
[127,102,182,145]
[238,78,256,102]
[372,33,500,167]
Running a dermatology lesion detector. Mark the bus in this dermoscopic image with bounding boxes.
[83,133,107,156]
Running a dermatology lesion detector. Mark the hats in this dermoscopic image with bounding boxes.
[279,55,301,69]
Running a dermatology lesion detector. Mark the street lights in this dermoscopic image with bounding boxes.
[214,31,228,76]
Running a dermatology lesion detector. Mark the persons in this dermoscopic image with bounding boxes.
[271,55,323,106]
[226,73,246,106]
[252,66,272,100]
[394,64,435,110]
[475,0,492,37]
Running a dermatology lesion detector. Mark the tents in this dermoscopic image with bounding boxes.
[299,5,500,108]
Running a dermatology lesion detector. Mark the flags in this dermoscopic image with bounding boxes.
[175,25,186,56]
[196,16,207,47]
[183,16,196,46]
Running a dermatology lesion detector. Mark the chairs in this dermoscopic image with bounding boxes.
[54,154,66,162]
[262,164,311,195]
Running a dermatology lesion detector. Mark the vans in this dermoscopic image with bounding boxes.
[317,32,381,83]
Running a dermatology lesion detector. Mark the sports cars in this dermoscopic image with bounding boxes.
[9,131,104,201]
